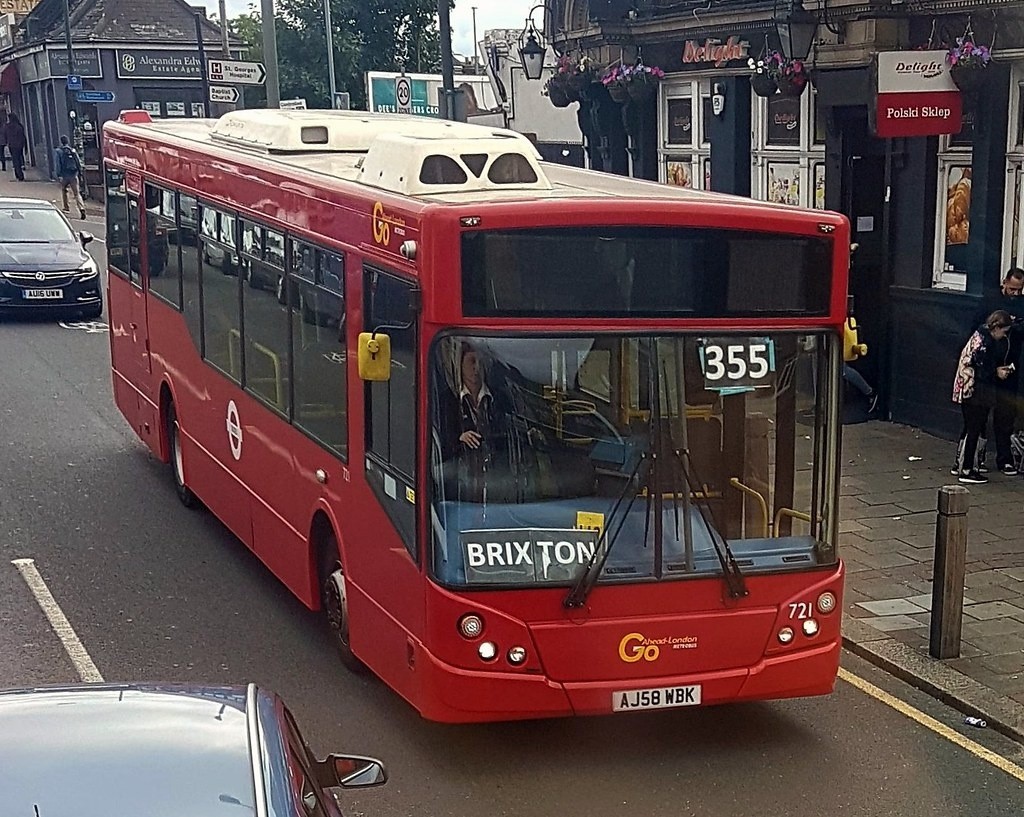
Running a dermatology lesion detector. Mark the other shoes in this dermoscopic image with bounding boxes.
[1000,463,1017,475]
[81,211,86,220]
[62,209,69,213]
[978,462,987,472]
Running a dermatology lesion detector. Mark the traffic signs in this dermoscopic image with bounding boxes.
[205,57,268,104]
[75,90,116,103]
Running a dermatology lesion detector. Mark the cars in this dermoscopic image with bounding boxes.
[108,201,170,276]
[0,196,103,318]
[0,683,388,817]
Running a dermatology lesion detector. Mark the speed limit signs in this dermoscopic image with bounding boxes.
[395,77,412,109]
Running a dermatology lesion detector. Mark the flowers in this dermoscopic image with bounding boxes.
[748,48,806,87]
[540,54,666,98]
[947,36,992,69]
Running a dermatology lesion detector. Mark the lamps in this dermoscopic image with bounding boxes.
[516,5,570,81]
[770,0,848,62]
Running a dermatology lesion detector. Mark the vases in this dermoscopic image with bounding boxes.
[554,74,570,92]
[608,87,632,103]
[948,65,989,93]
[548,86,572,108]
[568,75,592,92]
[749,77,778,97]
[774,77,807,96]
[627,80,660,101]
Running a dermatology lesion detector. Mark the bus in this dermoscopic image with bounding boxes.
[100,108,867,723]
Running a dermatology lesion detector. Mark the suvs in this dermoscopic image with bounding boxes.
[200,207,246,276]
[245,223,302,303]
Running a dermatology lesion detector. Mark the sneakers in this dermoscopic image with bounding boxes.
[950,465,958,475]
[866,387,878,413]
[958,469,988,483]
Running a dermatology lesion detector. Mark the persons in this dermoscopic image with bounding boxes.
[950,268,1024,484]
[55,135,86,220]
[799,244,879,419]
[0,112,29,183]
[434,336,510,465]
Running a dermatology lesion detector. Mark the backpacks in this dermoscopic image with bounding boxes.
[60,148,78,172]
